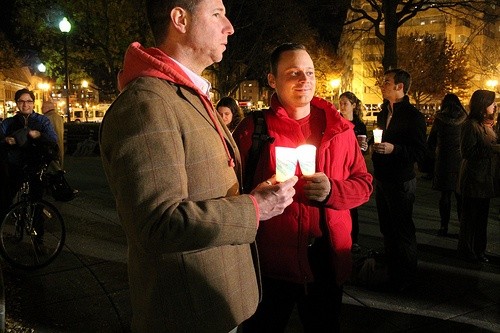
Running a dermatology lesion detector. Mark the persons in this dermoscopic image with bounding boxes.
[98,0,299,333]
[232,43,373,333]
[41,101,78,194]
[338,91,368,261]
[427,93,469,233]
[0,89,59,255]
[216,97,244,129]
[456,90,500,262]
[371,69,427,294]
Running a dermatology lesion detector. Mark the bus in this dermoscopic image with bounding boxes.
[72,104,110,123]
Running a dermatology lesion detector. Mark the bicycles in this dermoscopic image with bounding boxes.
[0,153,70,270]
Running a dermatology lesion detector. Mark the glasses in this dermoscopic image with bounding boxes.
[17,99,34,104]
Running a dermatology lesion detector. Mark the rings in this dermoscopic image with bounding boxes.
[266,180,271,185]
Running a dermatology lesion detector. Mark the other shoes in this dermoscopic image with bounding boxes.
[439,224,448,235]
[35,244,49,255]
[352,241,359,252]
[378,278,410,292]
[458,253,480,265]
[476,253,488,264]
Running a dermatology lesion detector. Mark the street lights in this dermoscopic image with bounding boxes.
[82,80,88,122]
[38,63,46,103]
[59,16,72,126]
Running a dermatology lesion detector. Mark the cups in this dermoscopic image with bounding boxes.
[357,134,366,150]
[274,146,298,183]
[296,144,317,177]
[373,128,383,144]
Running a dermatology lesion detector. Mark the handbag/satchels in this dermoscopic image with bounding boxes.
[52,169,78,201]
